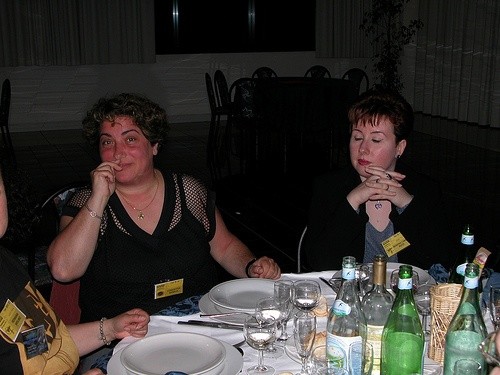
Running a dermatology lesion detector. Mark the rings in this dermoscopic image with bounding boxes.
[385,185,389,190]
[386,173,392,180]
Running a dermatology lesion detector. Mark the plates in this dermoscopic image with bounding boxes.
[209,277,292,311]
[365,261,430,289]
[286,326,326,363]
[198,293,293,325]
[107,339,245,374]
[120,332,225,375]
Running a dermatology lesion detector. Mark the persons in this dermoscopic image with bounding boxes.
[277,89,465,272]
[46,93,281,375]
[0,170,150,375]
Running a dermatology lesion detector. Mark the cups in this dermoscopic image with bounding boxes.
[348,341,373,374]
[310,345,347,375]
[453,359,482,375]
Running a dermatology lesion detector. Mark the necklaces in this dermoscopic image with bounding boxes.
[126,176,159,221]
[375,200,382,209]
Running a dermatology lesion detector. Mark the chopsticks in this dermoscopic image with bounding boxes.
[178,319,243,330]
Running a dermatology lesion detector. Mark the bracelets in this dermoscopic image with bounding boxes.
[100,317,111,345]
[85,202,102,219]
[246,258,258,278]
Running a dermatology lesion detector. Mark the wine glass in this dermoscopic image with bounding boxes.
[255,296,286,359]
[273,280,295,341]
[243,315,277,375]
[290,281,321,330]
[295,310,317,375]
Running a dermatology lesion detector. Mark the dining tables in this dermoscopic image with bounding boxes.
[107,265,445,375]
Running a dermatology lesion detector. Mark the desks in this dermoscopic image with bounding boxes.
[234,80,358,125]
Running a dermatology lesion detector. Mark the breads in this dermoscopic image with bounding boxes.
[303,331,326,360]
[312,301,328,317]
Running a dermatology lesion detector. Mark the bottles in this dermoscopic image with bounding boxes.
[450,223,476,283]
[380,264,425,375]
[444,263,488,375]
[326,256,366,374]
[360,255,396,374]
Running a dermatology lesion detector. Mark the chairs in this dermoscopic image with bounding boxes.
[29,184,84,305]
[204,66,370,183]
